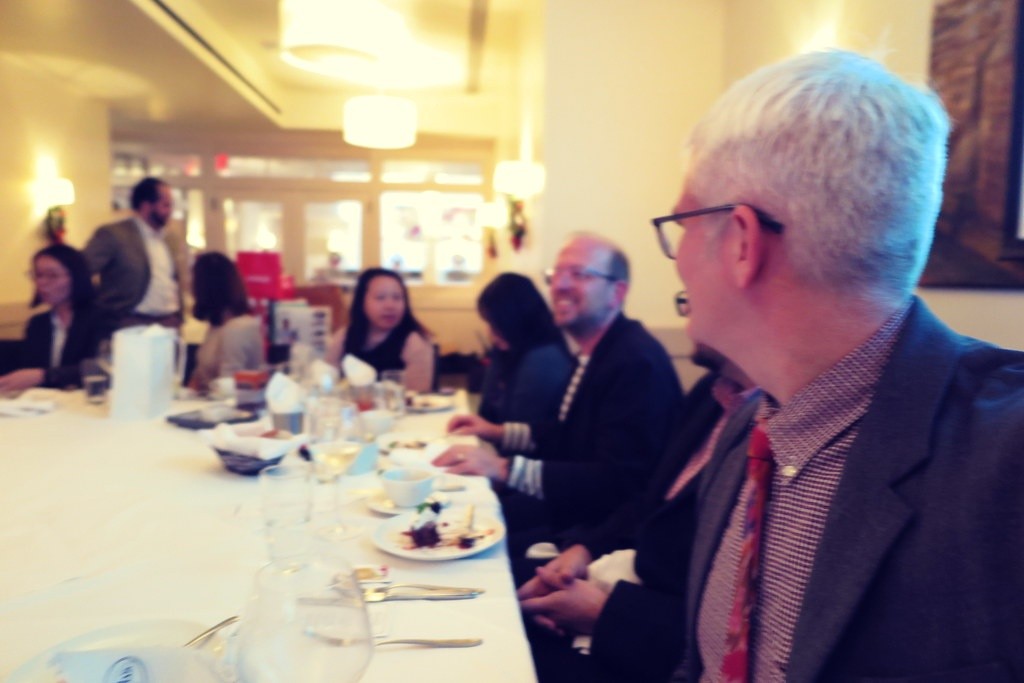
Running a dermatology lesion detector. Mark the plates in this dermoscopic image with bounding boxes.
[3,619,225,683]
[370,507,506,561]
[405,393,451,412]
[364,472,475,514]
[376,430,438,454]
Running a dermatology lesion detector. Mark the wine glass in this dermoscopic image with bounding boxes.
[256,464,319,612]
[305,397,363,541]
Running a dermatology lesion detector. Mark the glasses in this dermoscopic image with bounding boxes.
[673,291,694,317]
[541,261,619,287]
[28,267,68,282]
[652,205,784,262]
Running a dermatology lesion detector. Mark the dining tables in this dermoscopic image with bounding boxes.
[0,389,541,683]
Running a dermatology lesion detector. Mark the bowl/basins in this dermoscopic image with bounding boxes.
[208,423,295,476]
[381,467,436,508]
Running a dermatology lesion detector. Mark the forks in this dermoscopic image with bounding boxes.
[301,627,482,649]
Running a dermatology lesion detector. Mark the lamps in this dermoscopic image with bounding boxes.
[31,179,76,247]
[475,201,505,258]
[493,157,544,252]
[274,0,403,85]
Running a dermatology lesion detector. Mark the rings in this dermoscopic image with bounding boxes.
[458,453,463,458]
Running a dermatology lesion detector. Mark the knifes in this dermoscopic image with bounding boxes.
[341,591,480,602]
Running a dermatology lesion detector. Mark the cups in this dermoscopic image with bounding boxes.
[81,358,108,403]
[233,549,374,683]
[381,369,407,417]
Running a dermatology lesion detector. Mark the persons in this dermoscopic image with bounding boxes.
[515,287,762,683]
[0,242,100,391]
[477,272,581,424]
[326,268,434,392]
[188,251,263,393]
[81,177,185,358]
[432,232,683,570]
[277,318,296,344]
[652,48,1024,683]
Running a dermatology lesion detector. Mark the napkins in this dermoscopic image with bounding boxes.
[306,358,340,391]
[341,353,379,386]
[264,370,308,414]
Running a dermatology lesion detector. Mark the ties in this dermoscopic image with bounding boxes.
[719,424,773,683]
